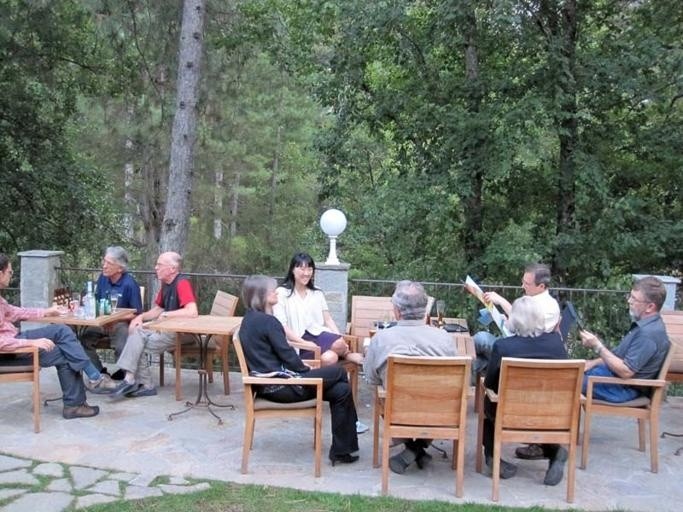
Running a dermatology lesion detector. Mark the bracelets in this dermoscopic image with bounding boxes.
[594,344,602,355]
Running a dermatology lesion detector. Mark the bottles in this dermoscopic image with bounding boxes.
[52,280,119,320]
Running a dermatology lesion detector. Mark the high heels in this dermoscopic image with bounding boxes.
[329,445,360,467]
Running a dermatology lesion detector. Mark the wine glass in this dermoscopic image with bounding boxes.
[382,310,390,330]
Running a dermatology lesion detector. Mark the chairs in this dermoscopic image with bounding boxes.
[92,285,145,350]
[576,334,679,474]
[659,310,682,384]
[230,323,326,481]
[0,340,48,435]
[370,351,478,499]
[287,330,363,414]
[156,289,242,400]
[552,301,578,347]
[474,356,586,504]
[342,292,410,367]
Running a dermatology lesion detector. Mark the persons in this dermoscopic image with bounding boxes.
[110,252,198,399]
[484,295,569,487]
[0,255,119,419]
[271,253,364,370]
[515,277,670,458]
[241,275,360,467]
[363,281,460,474]
[79,247,143,380]
[472,263,561,377]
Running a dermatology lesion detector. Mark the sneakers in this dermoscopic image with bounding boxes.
[356,420,369,434]
[111,370,126,380]
[63,402,99,419]
[485,454,517,480]
[126,384,158,398]
[109,380,138,400]
[86,376,122,394]
[515,443,551,461]
[543,447,568,486]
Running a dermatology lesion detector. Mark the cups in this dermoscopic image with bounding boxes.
[435,300,444,325]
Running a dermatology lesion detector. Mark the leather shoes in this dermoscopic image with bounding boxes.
[416,450,433,470]
[386,444,422,474]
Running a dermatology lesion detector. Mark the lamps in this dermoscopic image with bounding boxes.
[318,208,350,265]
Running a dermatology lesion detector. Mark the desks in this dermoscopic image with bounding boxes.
[32,304,133,413]
[144,315,246,427]
[369,315,474,361]
